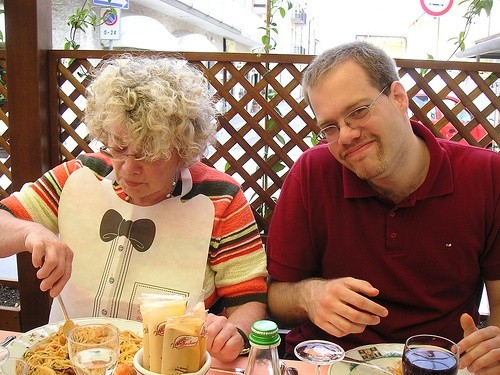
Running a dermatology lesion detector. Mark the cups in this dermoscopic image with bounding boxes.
[68,323,120,375]
[132,347,211,375]
[402,334,459,375]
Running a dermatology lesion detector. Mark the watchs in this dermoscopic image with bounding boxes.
[236,327,250,355]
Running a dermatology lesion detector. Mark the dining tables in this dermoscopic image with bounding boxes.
[0,329,332,375]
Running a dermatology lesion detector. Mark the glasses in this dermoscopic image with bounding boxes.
[316,85,387,147]
[98,131,162,161]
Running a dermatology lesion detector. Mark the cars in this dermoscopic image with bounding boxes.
[407,94,494,151]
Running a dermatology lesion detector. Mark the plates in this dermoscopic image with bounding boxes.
[0,316,144,375]
[328,343,475,375]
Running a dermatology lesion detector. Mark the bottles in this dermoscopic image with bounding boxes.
[244,320,282,375]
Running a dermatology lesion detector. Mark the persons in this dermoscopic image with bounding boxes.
[0,53,267,363]
[267,42,500,375]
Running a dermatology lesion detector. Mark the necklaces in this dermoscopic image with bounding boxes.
[125,168,180,204]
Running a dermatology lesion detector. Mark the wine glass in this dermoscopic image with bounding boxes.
[294,340,345,375]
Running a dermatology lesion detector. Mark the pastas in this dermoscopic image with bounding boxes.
[387,361,403,375]
[15,323,146,375]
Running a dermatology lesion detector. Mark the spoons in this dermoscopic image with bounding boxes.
[40,258,75,341]
